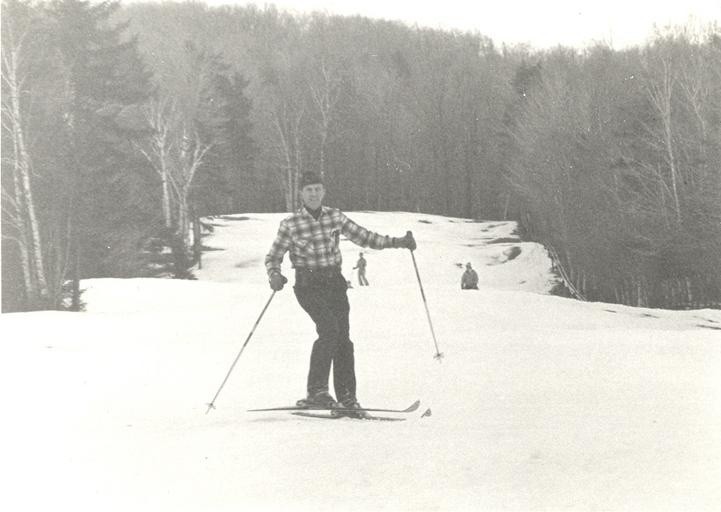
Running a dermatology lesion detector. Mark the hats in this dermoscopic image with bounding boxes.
[299,171,323,190]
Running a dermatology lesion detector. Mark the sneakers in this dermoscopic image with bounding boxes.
[307,384,335,407]
[332,399,365,417]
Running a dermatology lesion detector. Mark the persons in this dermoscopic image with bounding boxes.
[460,262,479,290]
[263,165,417,421]
[352,251,370,287]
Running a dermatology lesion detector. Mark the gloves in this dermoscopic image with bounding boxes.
[399,235,416,251]
[268,271,287,291]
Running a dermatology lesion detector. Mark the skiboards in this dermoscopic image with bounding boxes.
[250,402,430,422]
